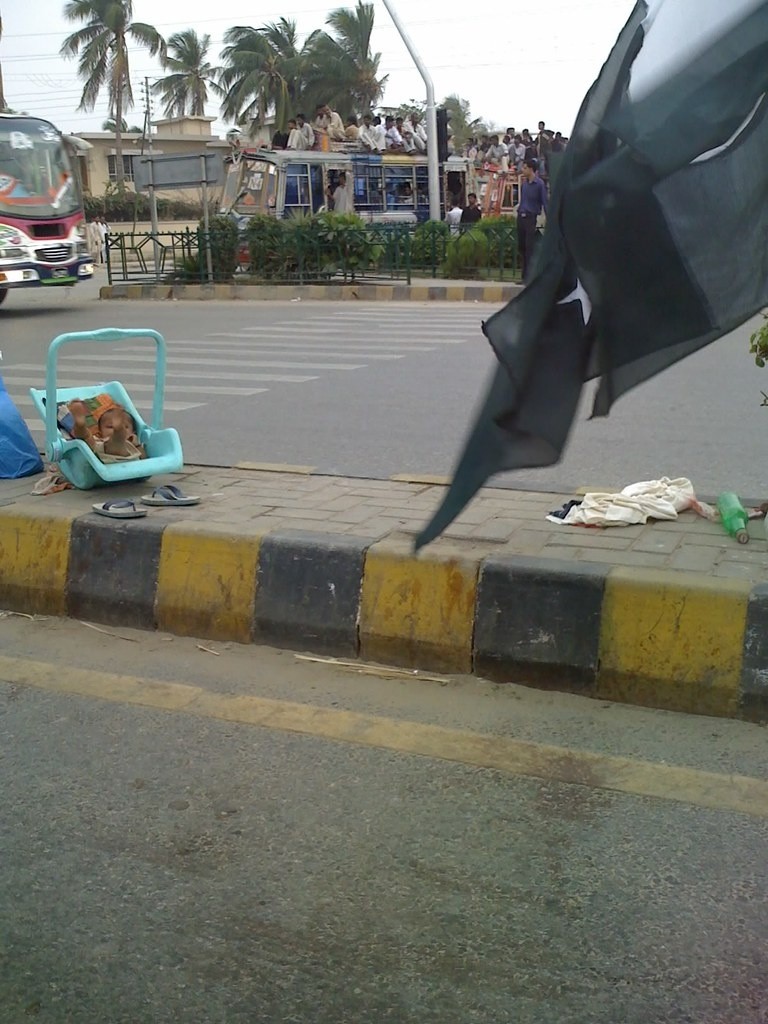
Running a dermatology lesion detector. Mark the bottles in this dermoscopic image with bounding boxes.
[715,491,749,544]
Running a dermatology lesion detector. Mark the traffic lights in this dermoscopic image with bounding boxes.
[438,108,454,163]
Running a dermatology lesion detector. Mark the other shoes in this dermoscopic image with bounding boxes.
[515,281,524,285]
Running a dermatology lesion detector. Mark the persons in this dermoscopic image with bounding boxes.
[88,216,111,265]
[275,104,569,284]
[66,397,145,467]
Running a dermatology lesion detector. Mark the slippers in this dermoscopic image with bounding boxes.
[141,485,200,505]
[92,498,147,517]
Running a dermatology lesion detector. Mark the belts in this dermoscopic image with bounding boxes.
[518,212,540,217]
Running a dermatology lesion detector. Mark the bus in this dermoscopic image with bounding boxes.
[481,172,554,234]
[0,113,94,285]
[216,151,480,249]
